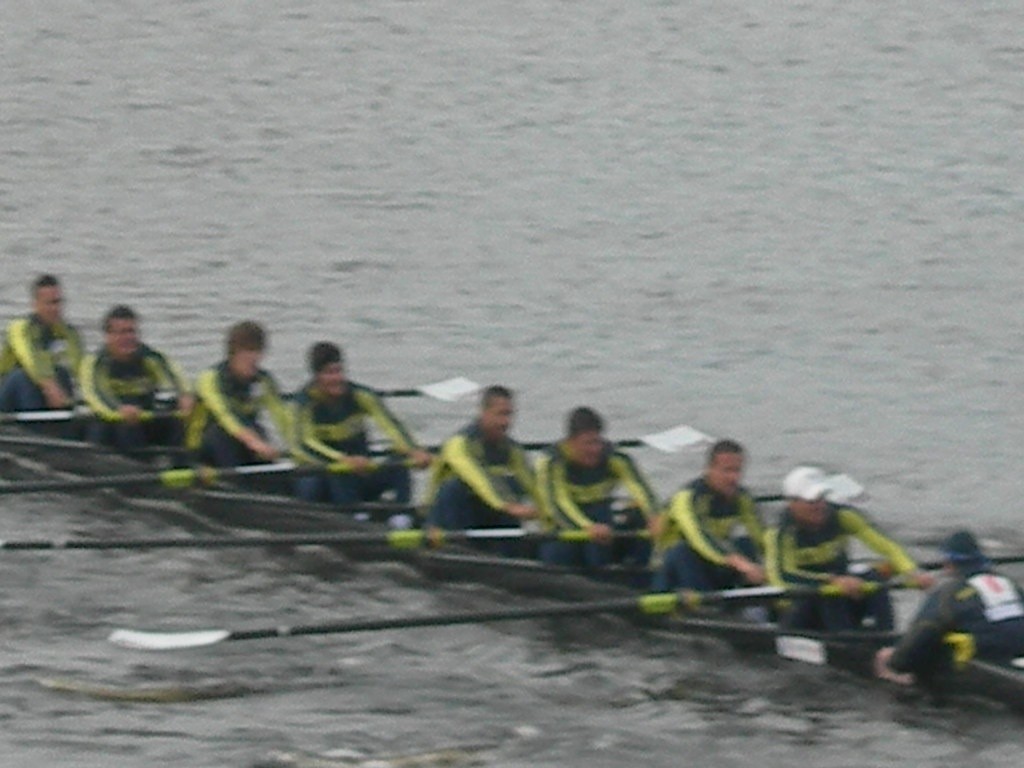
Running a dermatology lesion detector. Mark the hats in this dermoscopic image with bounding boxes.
[938,531,985,562]
[783,466,831,502]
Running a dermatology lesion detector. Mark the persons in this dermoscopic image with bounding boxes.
[419,386,536,549]
[649,439,768,597]
[761,466,925,631]
[535,407,660,569]
[185,321,286,494]
[79,305,192,462]
[0,273,85,411]
[277,340,429,524]
[886,532,1024,675]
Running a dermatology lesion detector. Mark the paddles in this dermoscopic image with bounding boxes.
[612,462,829,517]
[869,550,1023,574]
[551,471,865,545]
[138,372,481,424]
[0,408,74,423]
[0,457,304,494]
[289,418,719,476]
[1,525,530,553]
[106,579,921,651]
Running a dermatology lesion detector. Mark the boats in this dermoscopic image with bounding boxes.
[1,410,1024,706]
